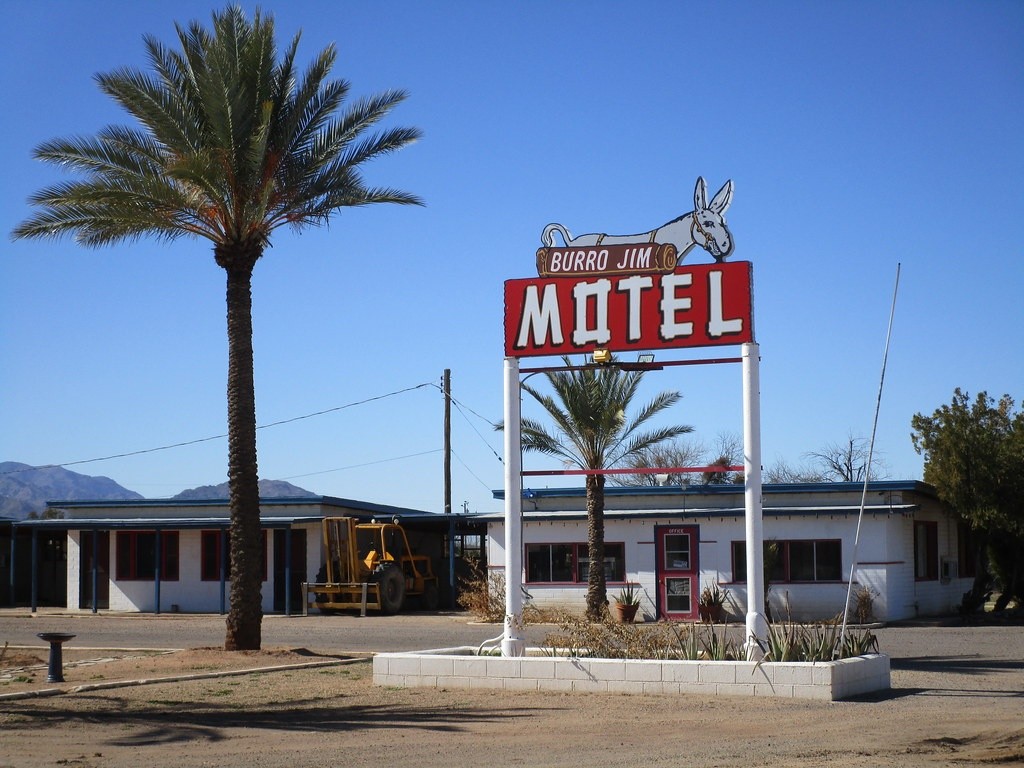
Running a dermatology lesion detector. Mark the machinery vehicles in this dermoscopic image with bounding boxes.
[280,514,439,618]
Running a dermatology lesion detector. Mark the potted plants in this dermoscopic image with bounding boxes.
[612,581,640,621]
[697,582,728,623]
